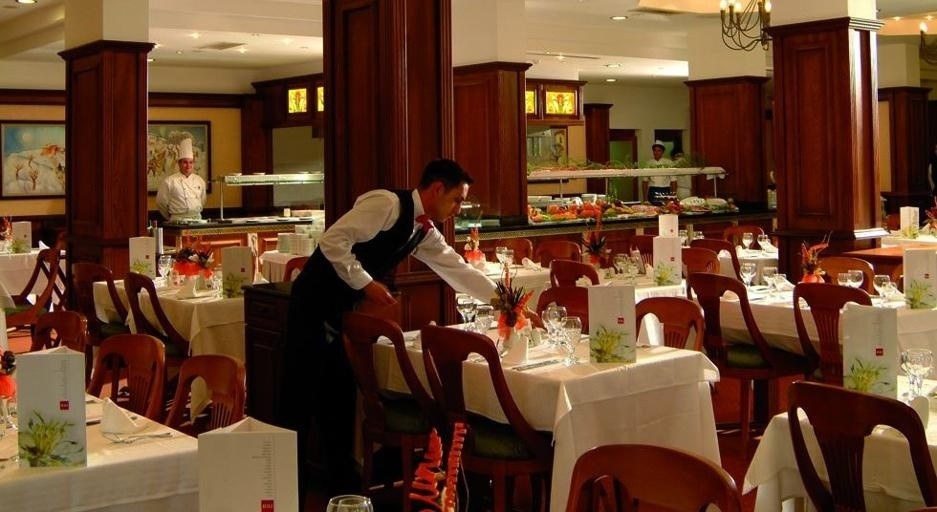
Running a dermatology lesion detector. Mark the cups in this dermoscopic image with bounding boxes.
[324,495,374,511]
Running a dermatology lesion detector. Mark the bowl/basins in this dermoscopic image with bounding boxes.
[274,223,326,257]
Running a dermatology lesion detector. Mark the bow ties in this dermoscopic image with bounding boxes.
[415,214,433,233]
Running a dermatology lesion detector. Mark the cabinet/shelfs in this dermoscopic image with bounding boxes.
[250,71,325,129]
[525,77,588,126]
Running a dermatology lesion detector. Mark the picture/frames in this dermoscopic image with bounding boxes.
[526,125,570,184]
[0,118,212,200]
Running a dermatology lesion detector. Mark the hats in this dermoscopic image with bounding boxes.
[177,138,194,160]
[655,140,664,145]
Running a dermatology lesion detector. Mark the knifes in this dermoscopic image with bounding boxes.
[86,416,138,426]
[514,357,565,372]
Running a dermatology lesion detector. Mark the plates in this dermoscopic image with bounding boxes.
[527,164,732,181]
[529,204,740,226]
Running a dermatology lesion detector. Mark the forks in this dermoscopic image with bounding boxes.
[102,432,173,443]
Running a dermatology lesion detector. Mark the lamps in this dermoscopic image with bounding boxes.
[919,22,937,65]
[718,0,772,53]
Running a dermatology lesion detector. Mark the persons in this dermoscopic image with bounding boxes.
[277,158,544,511]
[155,157,206,223]
[642,144,676,205]
[549,95,570,113]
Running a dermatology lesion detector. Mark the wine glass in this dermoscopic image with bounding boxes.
[739,262,898,309]
[898,348,934,405]
[610,252,642,285]
[542,306,584,366]
[456,293,495,336]
[156,254,223,296]
[494,245,513,273]
[5,399,19,460]
[673,229,772,260]
